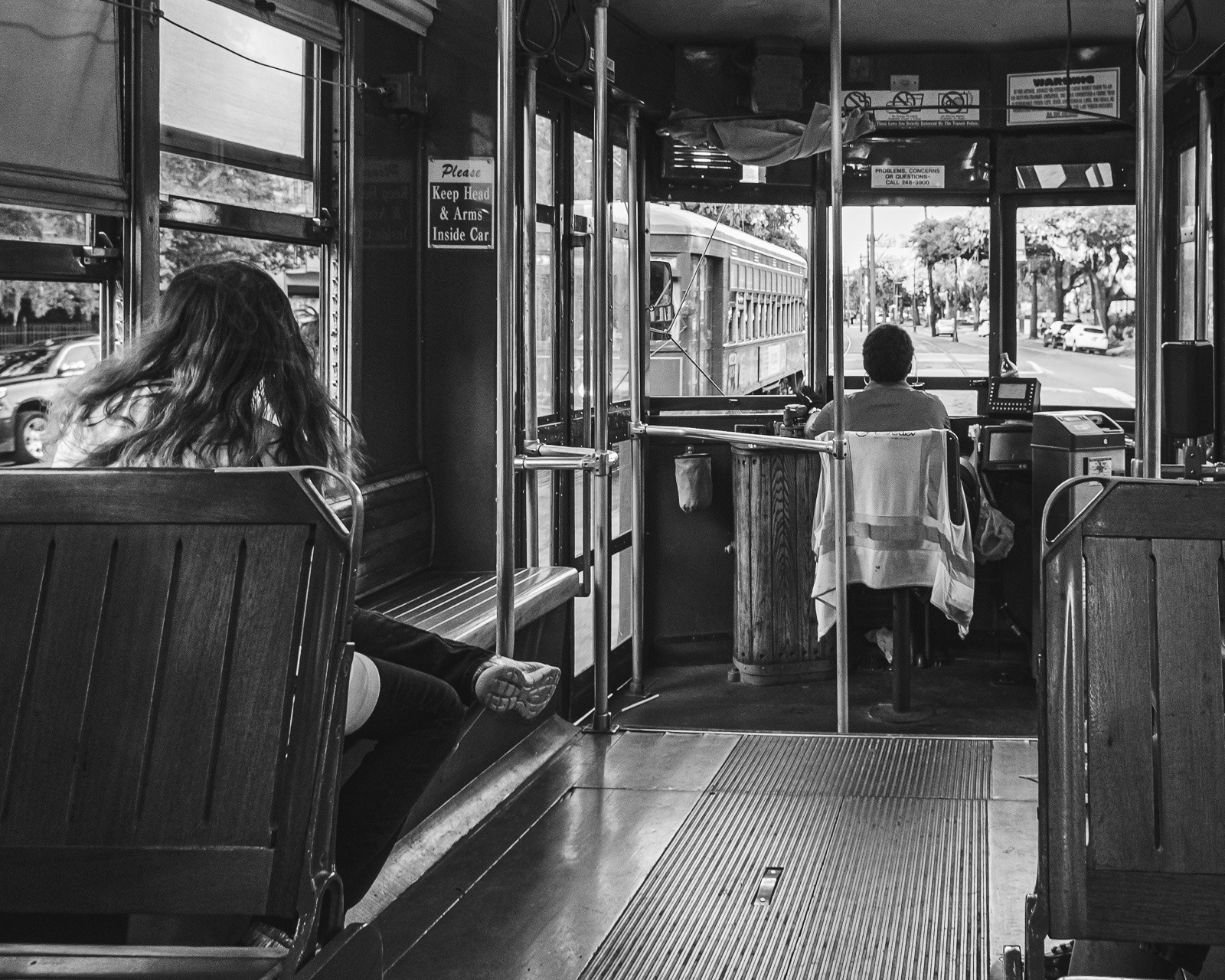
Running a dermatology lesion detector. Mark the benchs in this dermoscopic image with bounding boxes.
[0,463,388,979]
[1003,474,1225,980]
[356,567,579,647]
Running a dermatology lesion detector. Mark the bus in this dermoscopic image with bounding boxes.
[564,200,807,396]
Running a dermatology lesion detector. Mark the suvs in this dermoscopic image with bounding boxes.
[0,336,102,464]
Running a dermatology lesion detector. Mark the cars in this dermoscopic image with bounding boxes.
[1043,320,1080,348]
[977,321,992,336]
[934,319,955,337]
[1060,324,1111,354]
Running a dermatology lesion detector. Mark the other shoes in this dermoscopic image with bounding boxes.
[472,654,561,721]
[875,627,913,669]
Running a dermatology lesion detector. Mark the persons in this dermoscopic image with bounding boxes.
[39,262,560,911]
[804,324,982,666]
[1040,317,1047,336]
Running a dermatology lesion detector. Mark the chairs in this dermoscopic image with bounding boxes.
[812,428,975,725]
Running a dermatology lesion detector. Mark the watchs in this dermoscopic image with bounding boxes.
[808,406,822,414]
